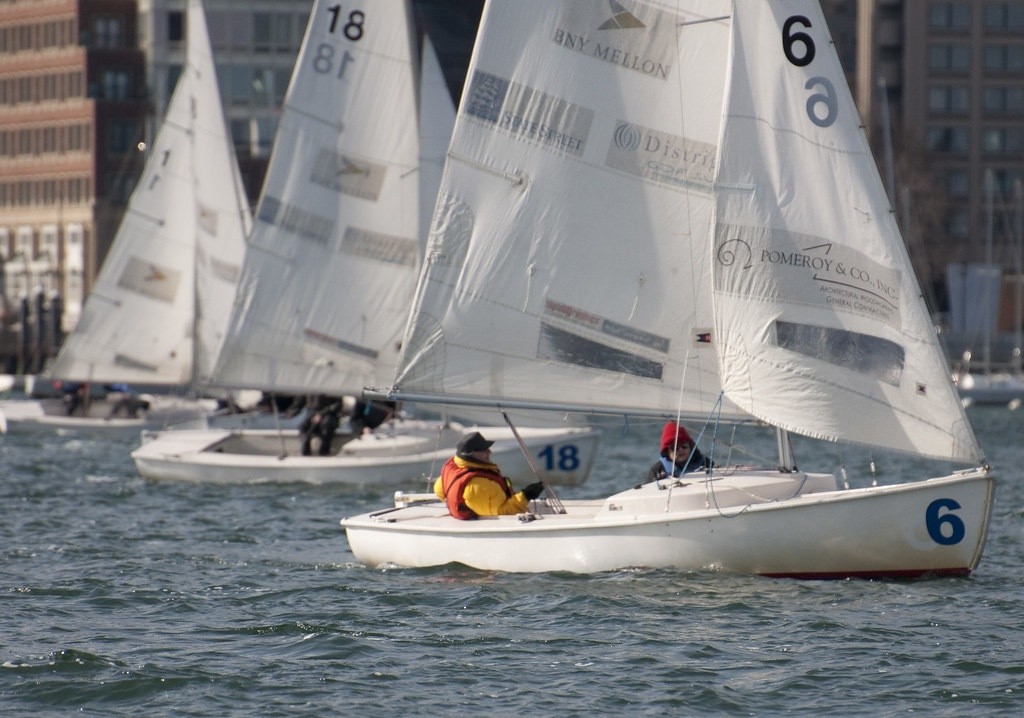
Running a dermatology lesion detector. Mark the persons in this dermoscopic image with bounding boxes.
[104,385,138,420]
[59,381,84,414]
[299,395,339,456]
[433,432,544,520]
[350,398,395,441]
[644,420,715,485]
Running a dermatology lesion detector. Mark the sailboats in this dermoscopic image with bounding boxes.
[127,1,603,487]
[341,0,999,578]
[32,0,255,430]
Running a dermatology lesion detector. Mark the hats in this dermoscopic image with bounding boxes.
[457,432,494,452]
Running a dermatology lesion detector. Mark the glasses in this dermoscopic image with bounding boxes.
[671,445,690,451]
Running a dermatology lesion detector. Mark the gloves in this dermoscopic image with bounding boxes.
[523,481,545,500]
